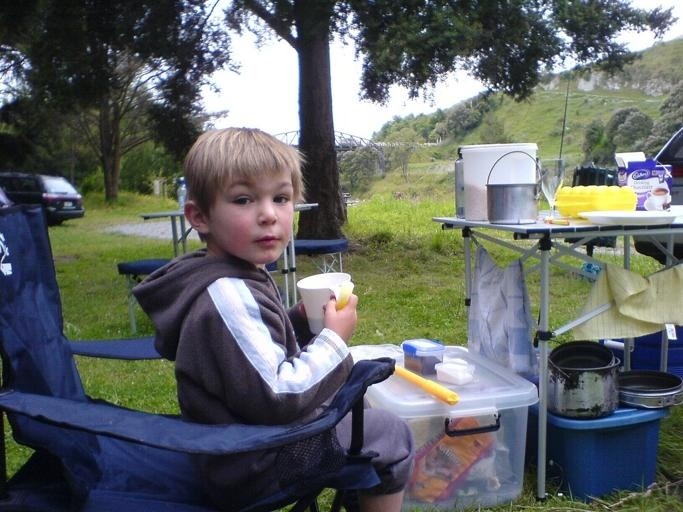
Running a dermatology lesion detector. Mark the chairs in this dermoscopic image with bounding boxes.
[0,203,396,512]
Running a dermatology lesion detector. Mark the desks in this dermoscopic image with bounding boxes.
[431,215,683,502]
[140,202,320,308]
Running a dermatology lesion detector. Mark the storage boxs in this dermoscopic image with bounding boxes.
[599,326,683,380]
[345,345,540,511]
[531,403,671,501]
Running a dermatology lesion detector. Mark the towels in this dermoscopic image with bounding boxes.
[466,244,542,386]
[575,262,682,342]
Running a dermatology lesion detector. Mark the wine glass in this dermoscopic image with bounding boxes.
[538,158,563,223]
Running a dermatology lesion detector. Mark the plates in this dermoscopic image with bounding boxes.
[644,199,670,211]
[579,211,675,224]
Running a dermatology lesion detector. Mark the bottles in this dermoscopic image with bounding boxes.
[177,176,187,214]
[455,148,464,219]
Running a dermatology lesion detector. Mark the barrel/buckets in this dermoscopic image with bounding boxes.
[486,150,543,224]
[460,143,539,222]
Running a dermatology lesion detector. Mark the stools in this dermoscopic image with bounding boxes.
[116,258,181,333]
[287,238,348,308]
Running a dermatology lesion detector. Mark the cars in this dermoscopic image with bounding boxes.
[0,171,86,226]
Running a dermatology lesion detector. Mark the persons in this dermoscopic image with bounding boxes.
[132,127,415,512]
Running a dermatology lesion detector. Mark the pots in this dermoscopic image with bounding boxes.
[545,348,620,419]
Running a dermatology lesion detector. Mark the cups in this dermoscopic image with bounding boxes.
[646,187,670,206]
[297,271,353,332]
